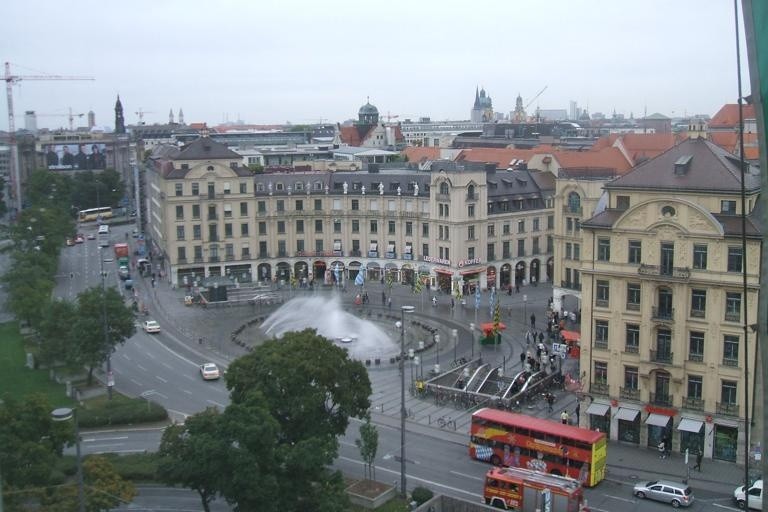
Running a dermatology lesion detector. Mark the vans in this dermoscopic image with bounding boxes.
[735,479,763,510]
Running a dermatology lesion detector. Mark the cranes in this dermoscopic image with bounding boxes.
[133,107,155,121]
[1,62,95,215]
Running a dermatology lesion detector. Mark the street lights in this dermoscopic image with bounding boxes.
[564,311,568,324]
[184,267,285,306]
[469,323,476,357]
[463,367,469,394]
[497,368,502,400]
[418,341,424,378]
[452,329,457,360]
[434,364,439,404]
[523,294,527,324]
[408,349,414,383]
[50,407,85,512]
[435,335,439,363]
[525,364,530,381]
[401,305,416,494]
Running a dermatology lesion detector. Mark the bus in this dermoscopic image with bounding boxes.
[79,206,112,222]
[98,225,111,246]
[484,465,589,512]
[470,408,606,487]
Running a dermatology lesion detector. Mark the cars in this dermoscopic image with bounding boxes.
[87,234,94,239]
[143,319,160,333]
[633,480,694,508]
[200,364,219,380]
[66,234,84,246]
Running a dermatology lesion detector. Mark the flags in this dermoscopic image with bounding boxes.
[334,261,501,336]
[289,272,296,289]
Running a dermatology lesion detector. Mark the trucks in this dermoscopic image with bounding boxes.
[114,244,130,278]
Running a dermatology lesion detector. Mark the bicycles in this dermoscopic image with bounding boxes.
[437,416,454,428]
[408,385,480,412]
[401,408,415,419]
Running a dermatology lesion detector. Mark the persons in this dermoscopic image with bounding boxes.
[45,144,106,171]
[561,410,568,425]
[657,439,666,460]
[693,449,703,472]
[298,274,318,289]
[515,297,577,413]
